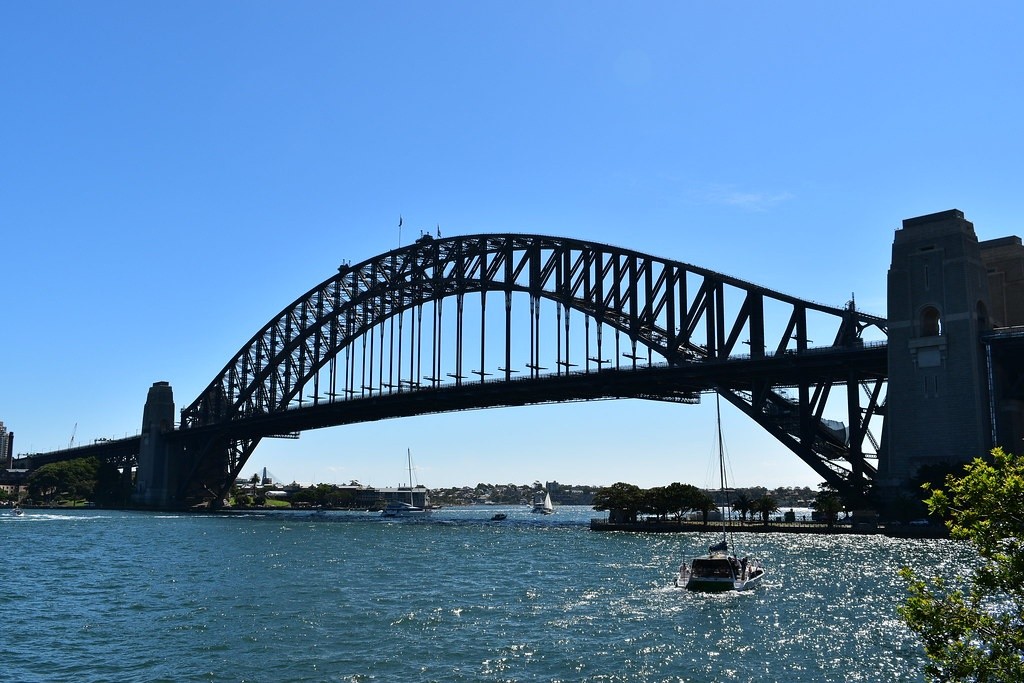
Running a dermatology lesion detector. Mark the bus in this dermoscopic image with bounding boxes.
[291,502,310,509]
[84,502,95,506]
[812,510,838,521]
[797,500,811,507]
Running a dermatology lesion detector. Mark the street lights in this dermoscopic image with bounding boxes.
[842,505,845,526]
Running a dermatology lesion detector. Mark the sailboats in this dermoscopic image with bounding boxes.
[542,492,554,514]
[685,389,766,593]
[382,448,434,516]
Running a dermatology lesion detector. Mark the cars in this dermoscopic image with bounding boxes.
[891,520,902,525]
[909,519,929,526]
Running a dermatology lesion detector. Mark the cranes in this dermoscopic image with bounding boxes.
[71,422,77,443]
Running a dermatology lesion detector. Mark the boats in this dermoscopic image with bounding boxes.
[808,500,820,509]
[12,506,25,517]
[491,514,507,520]
[310,512,326,517]
